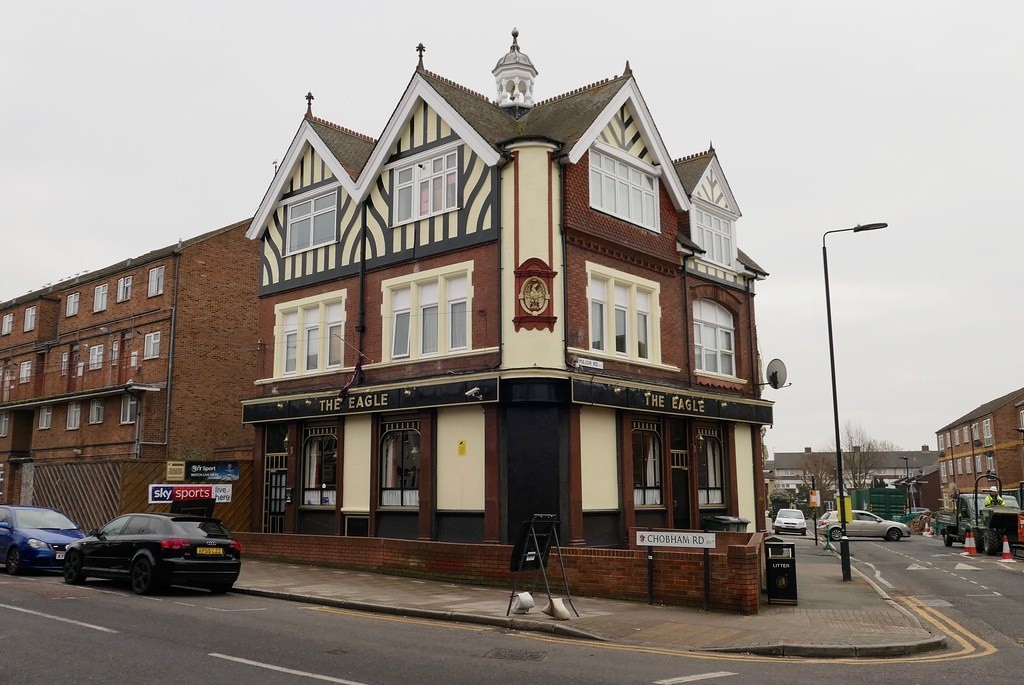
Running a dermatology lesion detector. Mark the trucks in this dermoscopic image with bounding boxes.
[936,494,1023,555]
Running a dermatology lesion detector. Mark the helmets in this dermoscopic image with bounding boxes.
[989,486,997,494]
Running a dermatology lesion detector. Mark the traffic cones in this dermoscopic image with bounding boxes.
[924,519,930,532]
[929,523,934,537]
[969,531,977,554]
[1001,535,1012,562]
[964,532,970,553]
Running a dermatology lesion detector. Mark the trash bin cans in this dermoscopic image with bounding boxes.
[764,541,797,605]
[737,519,751,532]
[702,514,742,532]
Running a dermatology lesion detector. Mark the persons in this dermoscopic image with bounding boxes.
[984,486,1006,508]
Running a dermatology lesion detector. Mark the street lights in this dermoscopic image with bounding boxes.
[821,222,889,582]
[898,457,911,514]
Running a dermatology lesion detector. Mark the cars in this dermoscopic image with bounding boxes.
[774,509,809,536]
[818,509,911,542]
[0,506,88,575]
[64,512,243,596]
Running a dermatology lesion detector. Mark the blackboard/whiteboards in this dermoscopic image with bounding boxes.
[509,520,561,573]
